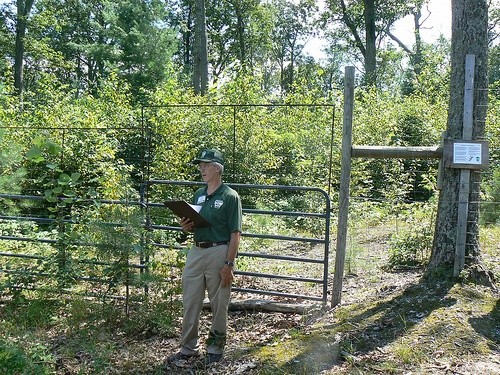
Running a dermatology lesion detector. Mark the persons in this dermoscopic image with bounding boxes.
[169,149,242,364]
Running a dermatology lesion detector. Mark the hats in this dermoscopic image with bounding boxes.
[192,148,225,164]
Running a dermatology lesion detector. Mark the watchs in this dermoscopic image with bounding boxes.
[224,261,234,266]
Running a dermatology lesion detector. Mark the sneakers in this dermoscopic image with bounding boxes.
[197,353,222,370]
[168,352,201,365]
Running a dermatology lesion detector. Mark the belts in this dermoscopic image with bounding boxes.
[194,241,227,249]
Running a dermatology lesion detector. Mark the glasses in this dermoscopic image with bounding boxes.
[197,164,215,170]
